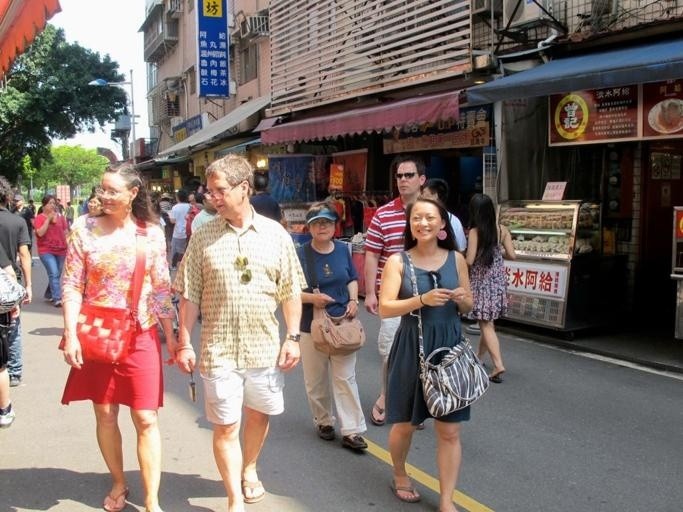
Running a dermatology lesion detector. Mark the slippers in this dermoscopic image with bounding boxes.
[369,395,387,426]
[393,478,420,503]
[241,479,266,504]
[103,486,129,512]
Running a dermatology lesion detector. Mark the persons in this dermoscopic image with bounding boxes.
[422,179,466,255]
[464,193,515,379]
[174,151,309,511]
[0,173,32,387]
[376,193,473,512]
[57,161,181,512]
[294,202,368,452]
[13,167,282,316]
[0,238,31,429]
[363,156,427,430]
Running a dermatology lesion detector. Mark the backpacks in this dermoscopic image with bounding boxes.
[186,206,200,241]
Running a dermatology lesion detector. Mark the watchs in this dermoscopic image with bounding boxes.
[286,332,300,343]
[348,298,359,306]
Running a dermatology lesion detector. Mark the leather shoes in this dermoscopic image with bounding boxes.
[318,425,335,440]
[342,436,367,447]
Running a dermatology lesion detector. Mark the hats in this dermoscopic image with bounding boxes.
[306,208,337,224]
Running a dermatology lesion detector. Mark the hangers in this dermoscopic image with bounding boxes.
[332,190,391,201]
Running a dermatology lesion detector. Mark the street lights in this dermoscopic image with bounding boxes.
[84,77,137,165]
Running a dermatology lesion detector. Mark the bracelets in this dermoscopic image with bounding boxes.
[418,294,425,306]
[177,345,195,354]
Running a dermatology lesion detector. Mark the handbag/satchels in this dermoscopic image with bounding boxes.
[311,288,366,354]
[419,334,490,419]
[58,303,143,364]
[0,264,27,314]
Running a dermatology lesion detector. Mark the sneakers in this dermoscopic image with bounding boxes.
[44,297,62,307]
[0,375,19,427]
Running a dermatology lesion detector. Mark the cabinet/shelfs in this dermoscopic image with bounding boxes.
[494,200,603,340]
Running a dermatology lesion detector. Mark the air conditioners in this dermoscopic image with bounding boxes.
[171,117,183,135]
[503,0,543,28]
[239,16,269,39]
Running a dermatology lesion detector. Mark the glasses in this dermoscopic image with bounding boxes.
[235,255,251,283]
[202,187,235,200]
[395,173,413,178]
[94,187,118,198]
